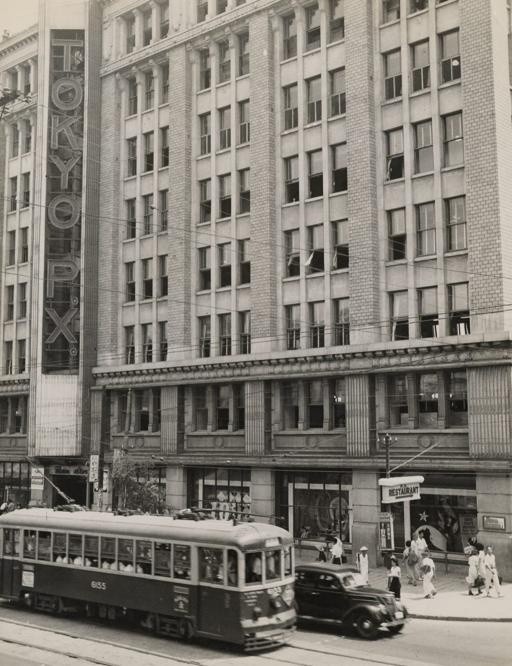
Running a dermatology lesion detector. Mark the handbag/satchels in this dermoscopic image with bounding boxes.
[475,575,485,586]
[422,565,431,573]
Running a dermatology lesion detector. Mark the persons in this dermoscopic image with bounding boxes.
[355,546,369,584]
[401,529,503,599]
[226,551,287,587]
[325,535,347,564]
[55,554,145,574]
[1,499,23,515]
[383,551,396,588]
[386,558,402,600]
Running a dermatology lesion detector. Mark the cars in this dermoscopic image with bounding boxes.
[290,561,408,641]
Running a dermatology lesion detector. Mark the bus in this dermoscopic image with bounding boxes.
[0,504,300,656]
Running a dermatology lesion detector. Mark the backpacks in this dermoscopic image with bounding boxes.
[406,548,418,565]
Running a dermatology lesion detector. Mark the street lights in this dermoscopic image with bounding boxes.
[375,432,399,568]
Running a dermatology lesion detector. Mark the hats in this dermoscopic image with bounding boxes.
[360,546,368,550]
